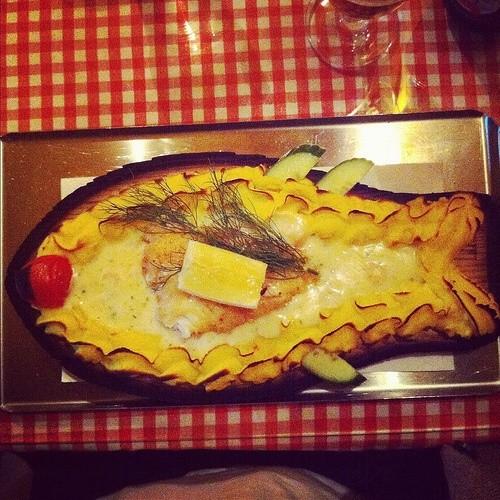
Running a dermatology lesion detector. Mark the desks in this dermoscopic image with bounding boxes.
[0,0,500,455]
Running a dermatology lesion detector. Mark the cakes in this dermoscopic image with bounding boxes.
[4,144,500,402]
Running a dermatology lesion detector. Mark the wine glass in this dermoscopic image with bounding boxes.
[304,0,405,68]
